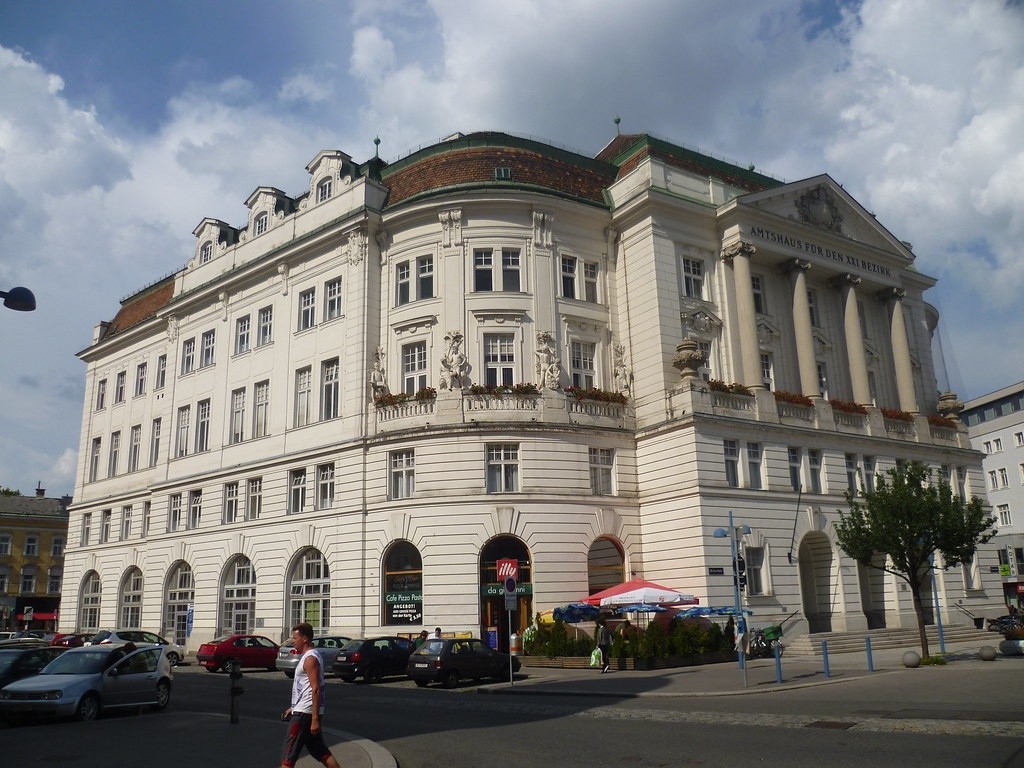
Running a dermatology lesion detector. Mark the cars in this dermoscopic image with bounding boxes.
[333,635,418,684]
[0,630,185,688]
[0,643,174,722]
[406,638,521,689]
[196,635,280,673]
[275,635,353,679]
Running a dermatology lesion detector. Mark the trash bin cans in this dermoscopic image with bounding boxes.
[511,633,523,656]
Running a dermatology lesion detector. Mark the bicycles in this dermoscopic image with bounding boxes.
[750,627,783,658]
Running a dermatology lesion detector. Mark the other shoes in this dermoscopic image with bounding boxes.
[603,665,609,672]
[600,671,608,673]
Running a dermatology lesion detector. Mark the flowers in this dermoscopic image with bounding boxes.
[468,382,541,394]
[708,379,752,397]
[926,415,956,430]
[830,399,867,413]
[774,390,813,406]
[879,406,916,422]
[375,387,437,407]
[564,384,627,405]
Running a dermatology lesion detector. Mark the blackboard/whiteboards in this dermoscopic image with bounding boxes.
[483,626,497,650]
[384,570,422,625]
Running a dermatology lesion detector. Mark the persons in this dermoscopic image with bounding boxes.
[614,358,632,392]
[614,620,636,671]
[15,627,23,638]
[1010,605,1018,619]
[371,361,386,403]
[535,345,561,391]
[280,623,340,768]
[438,346,466,390]
[597,620,613,673]
[429,627,443,653]
[415,630,428,649]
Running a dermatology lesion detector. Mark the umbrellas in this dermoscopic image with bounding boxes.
[538,602,622,641]
[581,577,699,638]
[618,604,668,633]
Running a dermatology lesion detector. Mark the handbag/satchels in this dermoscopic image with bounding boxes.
[590,648,604,668]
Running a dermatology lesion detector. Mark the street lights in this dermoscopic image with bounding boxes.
[713,511,752,690]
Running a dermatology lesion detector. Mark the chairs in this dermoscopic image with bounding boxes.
[319,640,326,647]
[328,641,334,648]
[460,645,469,654]
[248,638,256,647]
[375,645,388,650]
